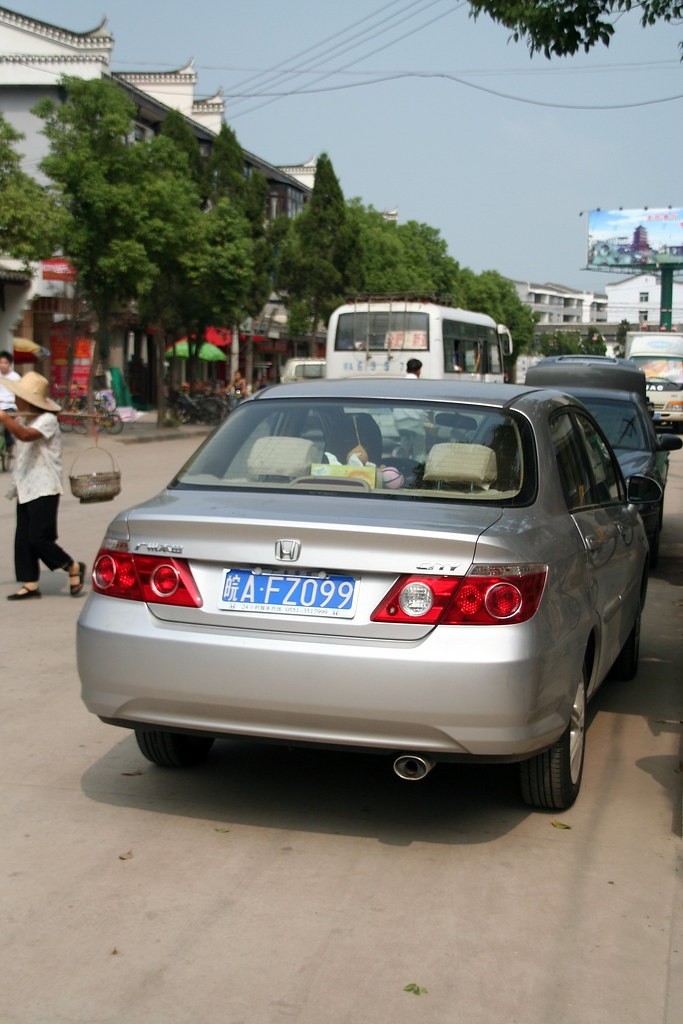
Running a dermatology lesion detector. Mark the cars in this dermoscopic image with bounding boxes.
[524,354,683,570]
[77,377,664,810]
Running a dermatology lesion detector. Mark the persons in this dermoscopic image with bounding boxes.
[0,351,21,458]
[325,414,383,468]
[446,353,462,371]
[222,371,248,411]
[390,359,432,464]
[504,371,512,384]
[0,372,85,600]
[260,377,267,388]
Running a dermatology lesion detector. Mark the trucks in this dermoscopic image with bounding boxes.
[625,331,683,434]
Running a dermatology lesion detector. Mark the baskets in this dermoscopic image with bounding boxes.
[70,447,122,504]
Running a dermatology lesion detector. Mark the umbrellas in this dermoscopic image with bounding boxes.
[163,341,227,360]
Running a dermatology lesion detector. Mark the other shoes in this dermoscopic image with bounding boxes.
[8,584,41,600]
[69,563,86,595]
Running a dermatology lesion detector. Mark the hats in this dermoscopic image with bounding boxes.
[0,370,64,412]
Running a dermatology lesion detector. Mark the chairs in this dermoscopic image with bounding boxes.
[248,434,321,482]
[421,442,498,497]
[323,412,384,463]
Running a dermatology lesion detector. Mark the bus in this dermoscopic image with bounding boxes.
[326,293,513,383]
